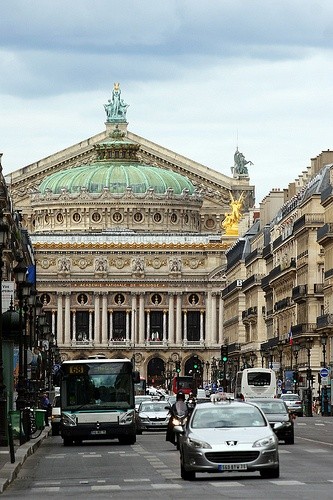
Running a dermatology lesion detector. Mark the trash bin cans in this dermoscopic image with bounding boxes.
[34,409,47,430]
[8,411,25,440]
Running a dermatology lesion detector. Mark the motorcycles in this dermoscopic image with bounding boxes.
[164,400,188,450]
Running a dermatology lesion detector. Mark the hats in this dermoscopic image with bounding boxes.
[41,394,47,397]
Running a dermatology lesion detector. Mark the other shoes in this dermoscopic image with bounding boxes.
[45,424,49,426]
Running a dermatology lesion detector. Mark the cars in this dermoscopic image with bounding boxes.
[178,393,279,482]
[51,394,60,434]
[243,396,295,444]
[136,400,173,433]
[280,393,303,416]
[134,386,238,418]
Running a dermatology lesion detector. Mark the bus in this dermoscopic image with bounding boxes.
[235,368,278,398]
[133,377,147,395]
[172,376,197,396]
[60,353,137,445]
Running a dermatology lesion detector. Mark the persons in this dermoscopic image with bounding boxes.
[210,392,217,402]
[168,390,192,416]
[42,394,49,426]
[187,392,196,405]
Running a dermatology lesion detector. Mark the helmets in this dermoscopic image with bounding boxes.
[176,391,185,401]
[189,392,193,398]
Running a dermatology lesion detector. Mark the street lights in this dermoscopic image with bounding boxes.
[292,341,300,396]
[0,220,61,465]
[305,334,314,418]
[206,345,274,390]
[277,341,284,391]
[320,331,330,416]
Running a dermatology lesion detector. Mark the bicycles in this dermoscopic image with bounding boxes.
[19,407,42,439]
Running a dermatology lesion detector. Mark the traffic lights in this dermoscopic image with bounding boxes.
[221,345,229,362]
[306,368,312,381]
[175,361,181,372]
[293,371,298,383]
[193,354,198,373]
